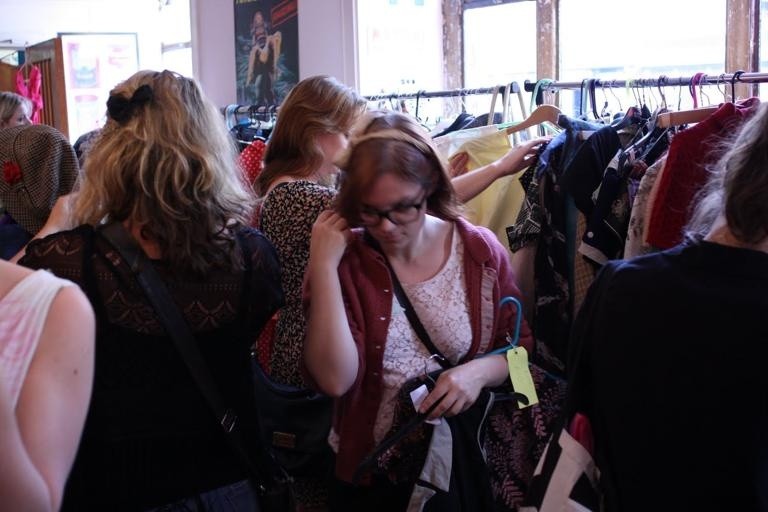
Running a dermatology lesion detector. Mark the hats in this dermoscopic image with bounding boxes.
[0,125,80,236]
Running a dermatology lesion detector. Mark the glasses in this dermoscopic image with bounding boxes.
[353,197,425,225]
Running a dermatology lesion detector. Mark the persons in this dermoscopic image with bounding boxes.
[0,124,96,512]
[9,69,288,512]
[256,76,554,391]
[297,111,528,512]
[0,91,35,130]
[563,100,768,512]
[238,10,287,105]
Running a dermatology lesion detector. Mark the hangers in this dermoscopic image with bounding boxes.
[226,104,280,130]
[18,46,33,83]
[362,297,533,465]
[359,83,502,127]
[508,71,746,163]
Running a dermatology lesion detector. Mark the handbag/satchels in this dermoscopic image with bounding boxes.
[250,455,298,512]
